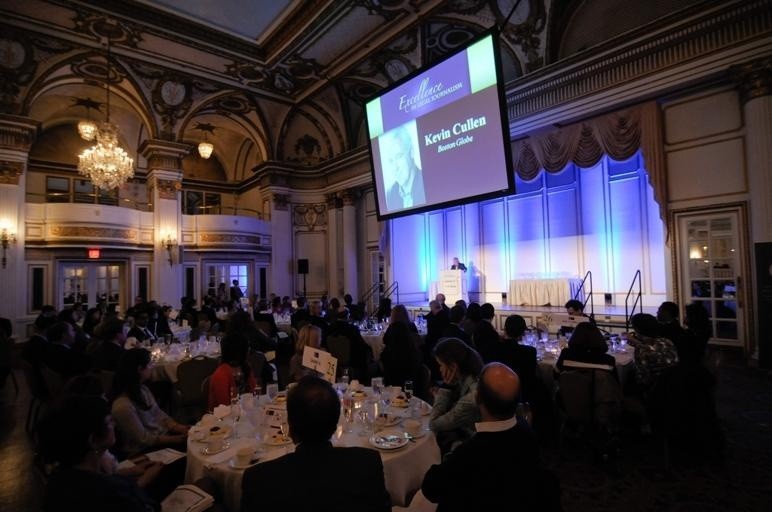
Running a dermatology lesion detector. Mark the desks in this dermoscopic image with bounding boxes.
[509,279,584,308]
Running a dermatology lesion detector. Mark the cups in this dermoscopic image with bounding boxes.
[151,336,218,359]
[235,449,251,466]
[358,316,428,332]
[209,427,225,453]
[522,333,627,360]
[403,420,421,434]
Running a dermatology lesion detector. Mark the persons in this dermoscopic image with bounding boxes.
[383,128,426,212]
[451,256,468,273]
[2,277,715,511]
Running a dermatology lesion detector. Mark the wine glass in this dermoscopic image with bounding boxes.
[232,376,414,437]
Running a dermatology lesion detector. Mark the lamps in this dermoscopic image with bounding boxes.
[78,30,135,193]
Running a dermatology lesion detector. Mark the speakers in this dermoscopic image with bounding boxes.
[298,258,308,274]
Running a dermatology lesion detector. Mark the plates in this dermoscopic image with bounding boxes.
[370,430,409,450]
[228,445,266,469]
[405,431,425,439]
